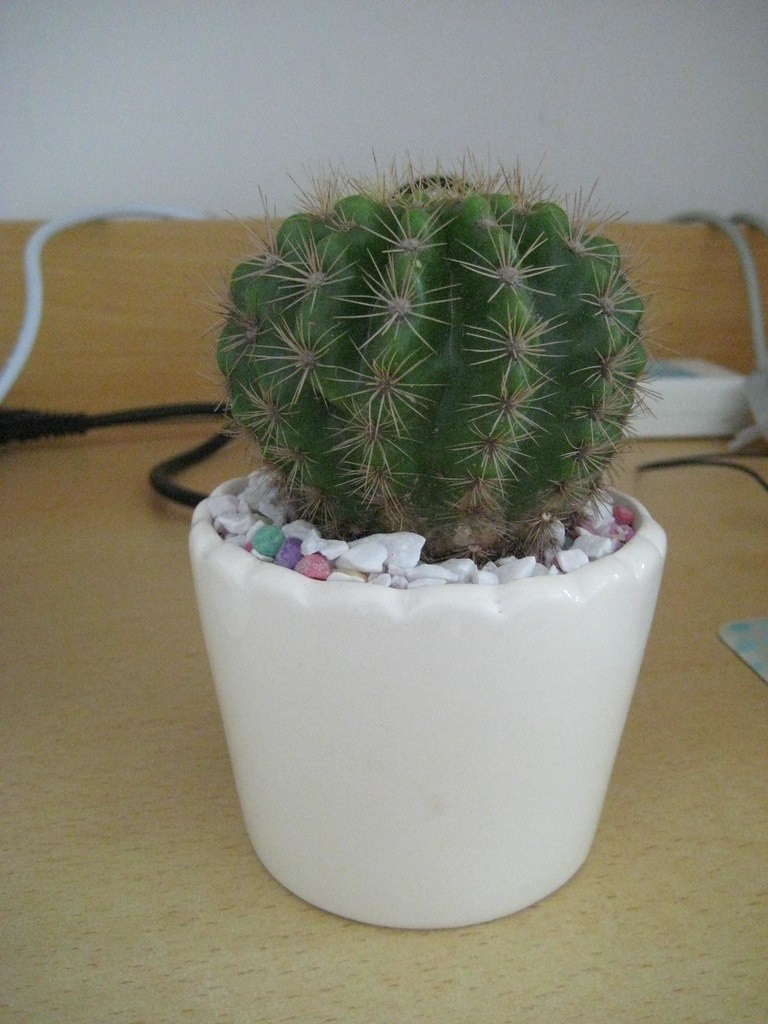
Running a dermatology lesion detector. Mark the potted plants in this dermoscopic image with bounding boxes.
[188,143,670,932]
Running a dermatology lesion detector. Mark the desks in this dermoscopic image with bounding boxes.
[1,211,768,1024]
